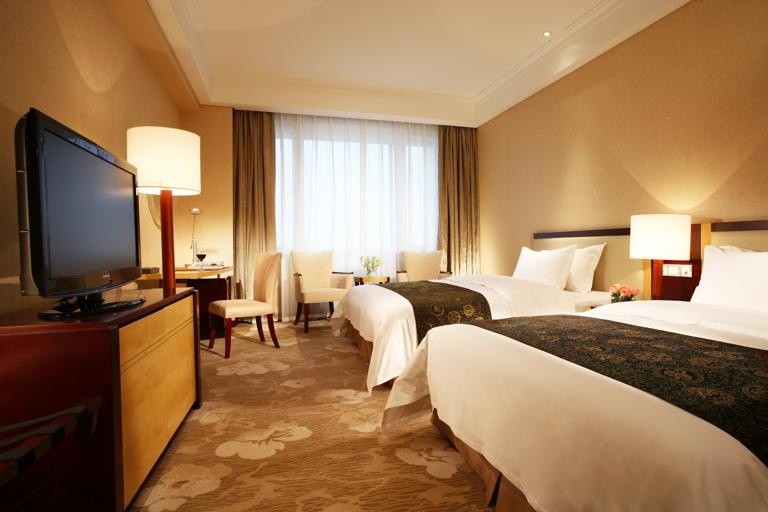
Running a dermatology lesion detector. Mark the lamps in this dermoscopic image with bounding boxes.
[186,208,202,267]
[127,126,201,288]
[629,214,691,299]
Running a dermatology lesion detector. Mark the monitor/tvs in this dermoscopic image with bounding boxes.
[14,105,147,320]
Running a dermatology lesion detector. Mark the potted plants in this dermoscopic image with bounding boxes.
[360,255,384,277]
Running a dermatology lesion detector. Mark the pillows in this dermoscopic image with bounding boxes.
[690,245,768,308]
[542,243,607,292]
[512,245,576,289]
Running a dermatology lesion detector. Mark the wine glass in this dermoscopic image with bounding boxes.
[196,245,206,274]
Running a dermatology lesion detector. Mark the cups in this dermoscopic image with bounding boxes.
[353,276,390,286]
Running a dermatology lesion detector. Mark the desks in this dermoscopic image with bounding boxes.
[136,267,231,279]
[1,290,202,512]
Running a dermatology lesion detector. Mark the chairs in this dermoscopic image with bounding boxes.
[396,250,452,282]
[290,249,354,334]
[207,251,283,359]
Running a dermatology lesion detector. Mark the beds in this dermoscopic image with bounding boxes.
[431,220,768,512]
[347,229,651,392]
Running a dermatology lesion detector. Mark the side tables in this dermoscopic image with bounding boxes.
[354,277,390,285]
